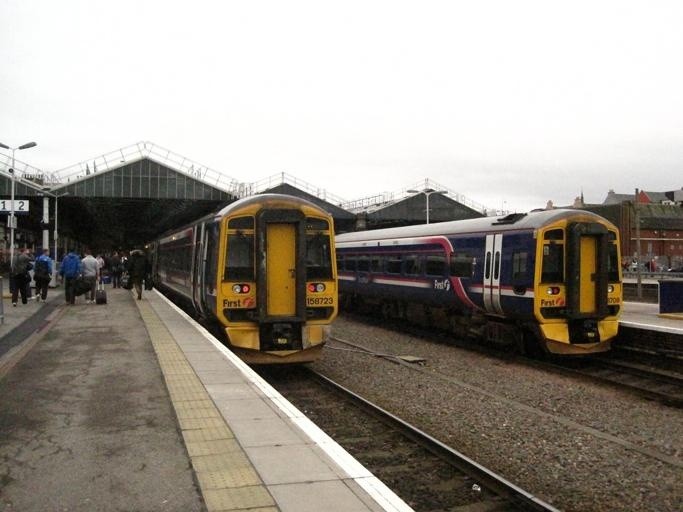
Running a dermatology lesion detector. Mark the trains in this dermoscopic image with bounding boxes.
[335,208,623,358]
[137,195,338,368]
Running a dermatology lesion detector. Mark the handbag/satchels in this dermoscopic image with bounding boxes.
[71,279,92,294]
[145,272,154,290]
[33,270,51,285]
[122,271,133,289]
[14,271,31,289]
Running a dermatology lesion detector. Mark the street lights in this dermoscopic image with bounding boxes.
[43,189,69,289]
[407,190,448,223]
[0,141,36,275]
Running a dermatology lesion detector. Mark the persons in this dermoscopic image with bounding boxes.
[0,239,154,307]
[621,251,658,278]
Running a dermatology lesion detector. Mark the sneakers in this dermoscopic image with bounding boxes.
[27,296,35,300]
[36,294,40,301]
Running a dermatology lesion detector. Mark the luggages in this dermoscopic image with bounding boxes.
[96,279,106,304]
